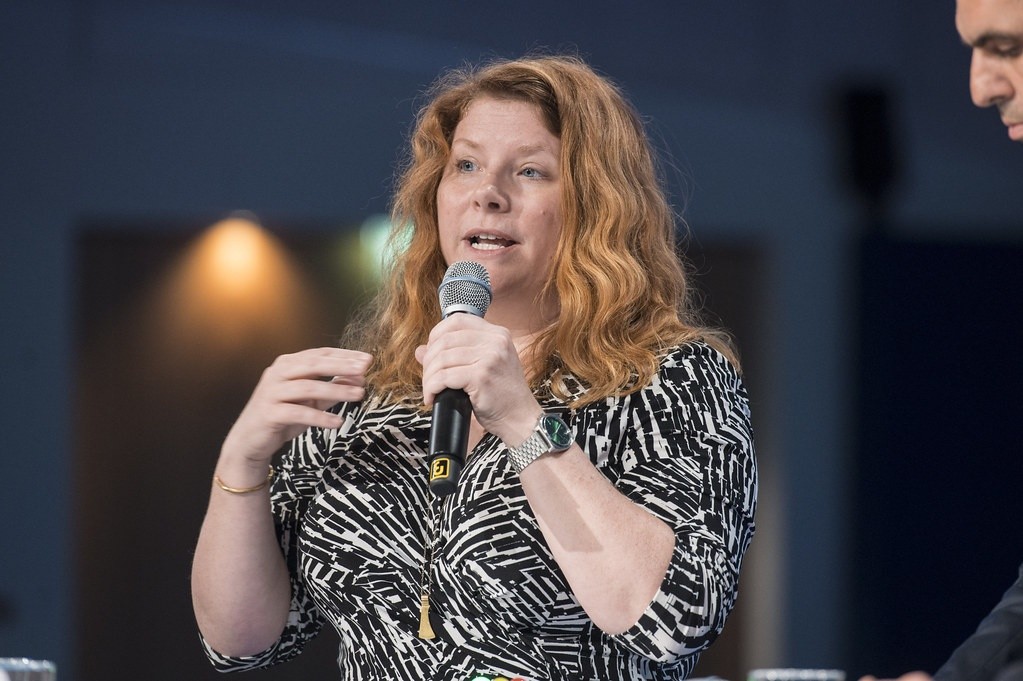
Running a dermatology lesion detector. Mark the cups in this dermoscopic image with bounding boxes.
[0,657,58,681]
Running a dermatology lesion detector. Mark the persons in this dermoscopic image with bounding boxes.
[861,1,1023,681]
[188,51,758,681]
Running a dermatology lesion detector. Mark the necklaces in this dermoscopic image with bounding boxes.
[417,495,450,639]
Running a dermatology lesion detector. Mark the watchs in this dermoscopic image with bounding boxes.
[506,412,576,473]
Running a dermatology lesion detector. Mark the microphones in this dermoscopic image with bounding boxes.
[426,261,494,497]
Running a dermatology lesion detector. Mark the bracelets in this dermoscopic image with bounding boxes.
[213,464,274,494]
